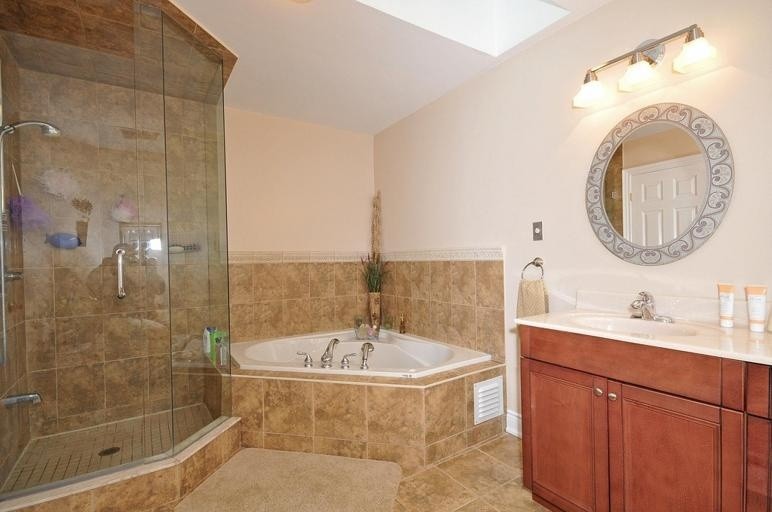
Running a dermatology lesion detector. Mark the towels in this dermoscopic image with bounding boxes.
[515,277,551,319]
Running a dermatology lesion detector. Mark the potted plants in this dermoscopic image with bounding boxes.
[360,253,391,329]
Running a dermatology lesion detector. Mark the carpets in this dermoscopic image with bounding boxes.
[171,448,404,511]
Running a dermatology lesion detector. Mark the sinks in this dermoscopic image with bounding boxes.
[561,311,701,344]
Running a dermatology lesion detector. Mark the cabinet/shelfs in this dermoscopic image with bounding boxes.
[746,362,772,512]
[517,325,748,512]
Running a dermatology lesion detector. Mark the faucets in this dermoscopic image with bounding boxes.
[630,293,655,316]
[2,393,43,407]
[321,337,341,365]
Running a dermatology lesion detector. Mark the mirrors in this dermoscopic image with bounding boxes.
[586,103,736,269]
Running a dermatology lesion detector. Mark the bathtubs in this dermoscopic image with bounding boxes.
[230,326,505,378]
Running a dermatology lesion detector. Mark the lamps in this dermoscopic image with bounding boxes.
[571,24,717,110]
